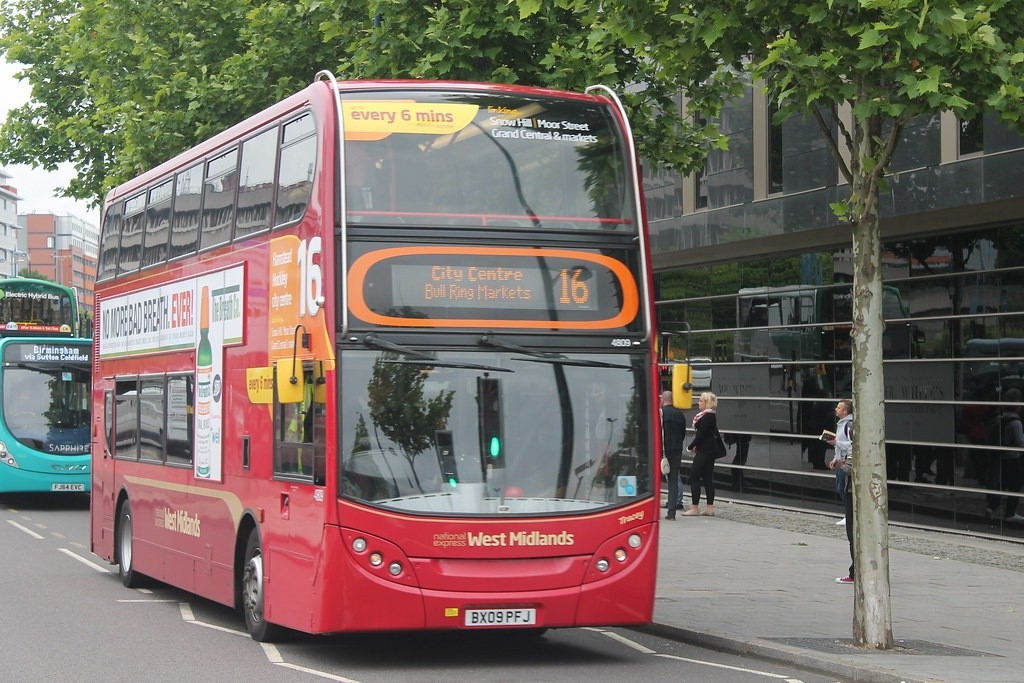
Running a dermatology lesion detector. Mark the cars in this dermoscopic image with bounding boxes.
[682,355,712,390]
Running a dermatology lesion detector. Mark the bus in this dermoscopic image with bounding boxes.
[88,68,695,643]
[0,276,82,341]
[1,335,93,498]
[730,284,927,443]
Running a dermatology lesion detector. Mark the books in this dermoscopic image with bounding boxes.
[819,430,836,442]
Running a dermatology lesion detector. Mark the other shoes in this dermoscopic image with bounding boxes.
[924,469,936,476]
[681,509,700,516]
[660,503,668,508]
[699,510,715,516]
[915,476,932,483]
[676,504,683,510]
[665,514,676,521]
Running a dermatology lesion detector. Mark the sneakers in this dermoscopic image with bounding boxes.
[835,517,846,526]
[1009,513,1024,521]
[984,508,993,522]
[835,576,854,584]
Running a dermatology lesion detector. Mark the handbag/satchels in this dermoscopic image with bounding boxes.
[834,469,848,497]
[710,426,727,459]
[660,456,671,476]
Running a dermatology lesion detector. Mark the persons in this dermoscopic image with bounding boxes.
[681,391,728,516]
[835,428,854,583]
[886,362,1024,522]
[826,400,854,526]
[658,391,685,520]
[722,431,751,488]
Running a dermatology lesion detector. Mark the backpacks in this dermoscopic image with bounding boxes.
[723,433,737,445]
[987,413,1021,456]
